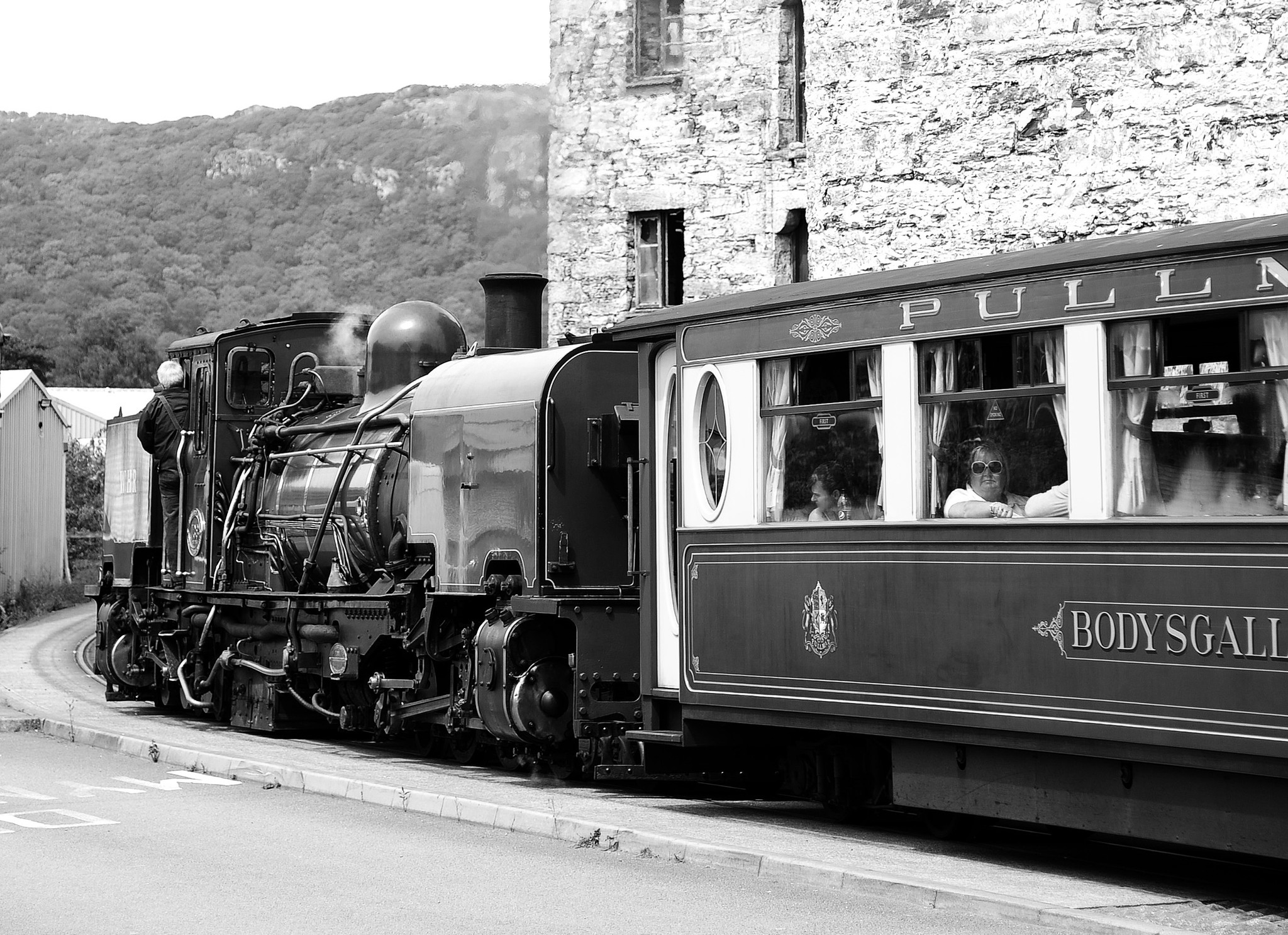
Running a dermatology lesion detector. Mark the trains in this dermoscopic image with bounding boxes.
[84,214,1288,866]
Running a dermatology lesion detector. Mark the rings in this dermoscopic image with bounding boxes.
[994,507,999,510]
[1003,510,1008,513]
[998,508,1003,511]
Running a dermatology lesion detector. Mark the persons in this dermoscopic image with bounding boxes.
[1024,480,1069,517]
[943,442,1030,518]
[808,461,884,521]
[137,360,191,589]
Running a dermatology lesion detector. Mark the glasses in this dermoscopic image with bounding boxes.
[970,460,1005,475]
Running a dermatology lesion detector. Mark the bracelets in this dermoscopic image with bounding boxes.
[989,502,1002,516]
